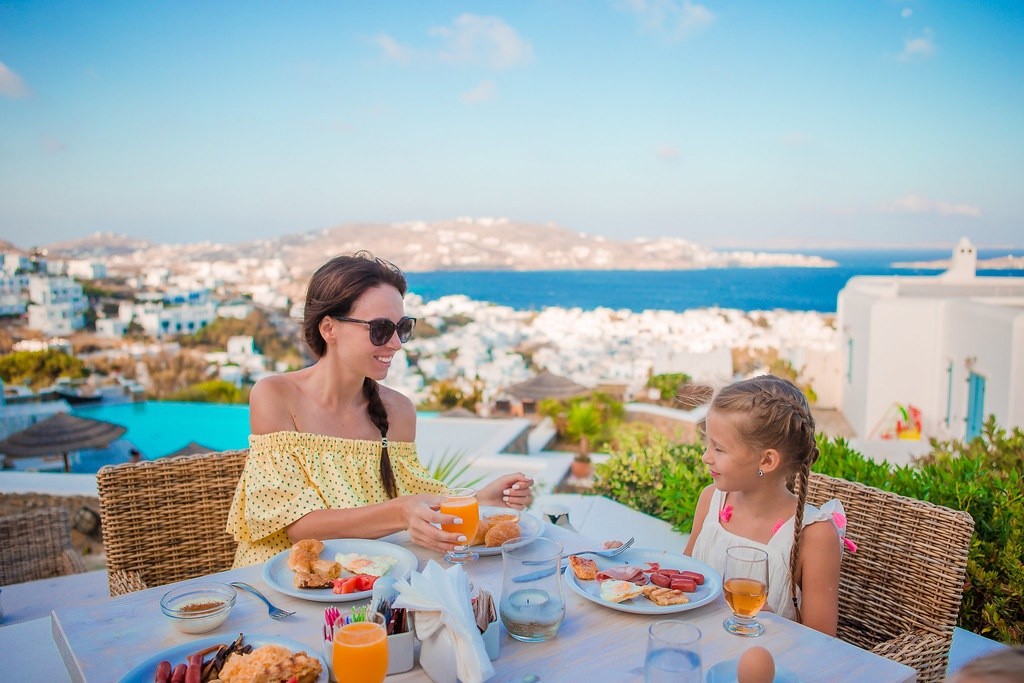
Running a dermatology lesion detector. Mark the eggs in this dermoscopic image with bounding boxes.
[334,552,398,577]
[601,579,644,603]
[738,646,775,683]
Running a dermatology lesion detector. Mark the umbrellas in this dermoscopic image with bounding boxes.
[0,411,128,474]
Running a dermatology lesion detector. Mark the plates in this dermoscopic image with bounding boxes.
[703,658,804,683]
[430,505,545,557]
[262,538,420,604]
[564,546,722,615]
[116,633,329,683]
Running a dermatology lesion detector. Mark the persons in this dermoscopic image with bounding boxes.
[683,374,858,637]
[226,250,535,572]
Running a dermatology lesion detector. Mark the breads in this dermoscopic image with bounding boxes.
[471,520,521,546]
[288,538,341,587]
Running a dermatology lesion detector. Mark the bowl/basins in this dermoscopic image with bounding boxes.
[482,507,521,528]
[160,582,237,634]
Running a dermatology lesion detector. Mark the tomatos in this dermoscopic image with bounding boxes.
[332,574,378,593]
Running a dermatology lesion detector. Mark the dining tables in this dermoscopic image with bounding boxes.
[51,506,917,682]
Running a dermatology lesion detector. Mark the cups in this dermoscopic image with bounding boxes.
[388,628,414,675]
[645,619,702,683]
[331,610,388,683]
[499,536,566,643]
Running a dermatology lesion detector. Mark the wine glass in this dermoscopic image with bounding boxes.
[723,546,769,638]
[439,487,480,564]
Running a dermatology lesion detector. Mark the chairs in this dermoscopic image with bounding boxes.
[96,450,250,598]
[793,469,975,683]
[0,507,85,587]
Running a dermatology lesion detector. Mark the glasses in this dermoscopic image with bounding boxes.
[330,315,417,346]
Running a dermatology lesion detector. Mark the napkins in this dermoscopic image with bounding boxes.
[390,556,496,683]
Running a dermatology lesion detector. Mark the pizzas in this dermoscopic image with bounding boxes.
[567,556,600,579]
[643,585,689,605]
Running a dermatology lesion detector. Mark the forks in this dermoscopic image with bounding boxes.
[231,582,296,621]
[522,536,634,566]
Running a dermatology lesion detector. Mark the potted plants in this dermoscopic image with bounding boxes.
[571,453,591,478]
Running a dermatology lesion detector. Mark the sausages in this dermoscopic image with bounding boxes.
[154,653,205,683]
[649,569,704,593]
